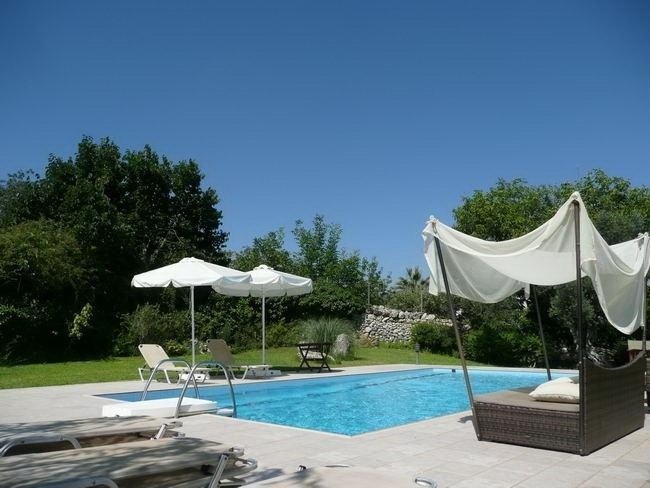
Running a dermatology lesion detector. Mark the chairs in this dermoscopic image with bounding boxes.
[137,338,272,386]
[1,415,259,488]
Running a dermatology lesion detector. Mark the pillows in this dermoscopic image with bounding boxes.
[528,376,579,404]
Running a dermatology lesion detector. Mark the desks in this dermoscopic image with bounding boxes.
[294,342,333,375]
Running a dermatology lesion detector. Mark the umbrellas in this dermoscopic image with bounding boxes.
[131,256,253,373]
[212,263,313,366]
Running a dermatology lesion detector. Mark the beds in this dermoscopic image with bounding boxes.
[471,350,645,458]
